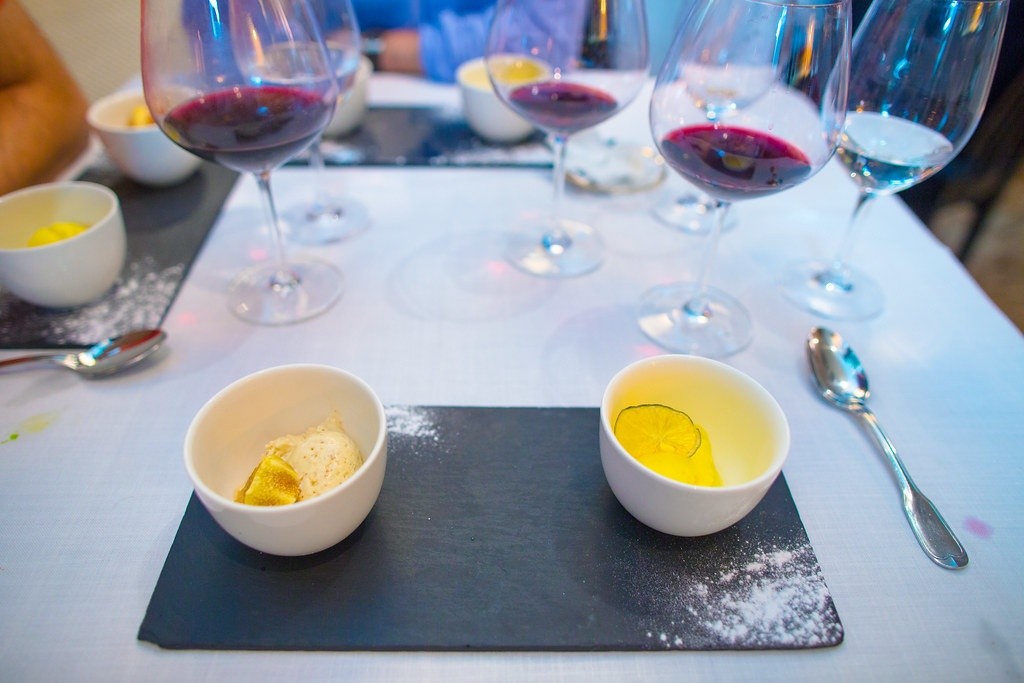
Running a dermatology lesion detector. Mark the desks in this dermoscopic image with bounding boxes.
[0,70,1023,683]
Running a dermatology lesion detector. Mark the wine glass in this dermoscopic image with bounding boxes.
[485,1,651,281]
[634,1,851,360]
[779,0,1011,323]
[648,0,797,234]
[139,0,347,326]
[229,0,371,246]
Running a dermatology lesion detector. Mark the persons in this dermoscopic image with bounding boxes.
[179,0,584,88]
[0,1,93,195]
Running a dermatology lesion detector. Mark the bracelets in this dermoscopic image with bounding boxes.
[363,30,380,73]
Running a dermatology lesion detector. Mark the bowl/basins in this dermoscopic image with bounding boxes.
[599,354,792,537]
[0,181,126,311]
[454,52,551,143]
[86,86,204,188]
[321,53,374,138]
[183,363,388,556]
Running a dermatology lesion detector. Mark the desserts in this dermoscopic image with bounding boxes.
[611,403,723,489]
[232,416,362,508]
[20,220,90,247]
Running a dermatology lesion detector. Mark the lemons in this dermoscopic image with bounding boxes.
[613,404,703,458]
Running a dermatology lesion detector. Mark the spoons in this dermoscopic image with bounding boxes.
[804,325,969,570]
[0,329,167,379]
